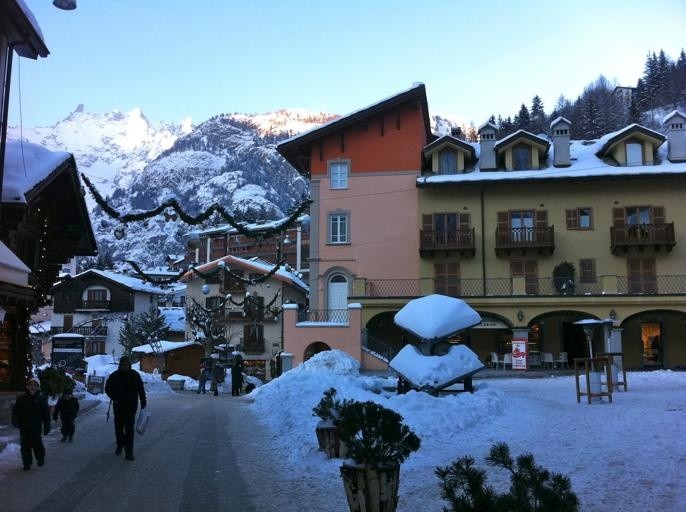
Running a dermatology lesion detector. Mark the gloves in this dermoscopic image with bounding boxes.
[141,401,146,409]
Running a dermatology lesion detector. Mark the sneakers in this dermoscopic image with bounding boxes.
[23,457,44,471]
[61,436,73,442]
[115,443,134,460]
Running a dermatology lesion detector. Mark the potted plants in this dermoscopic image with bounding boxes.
[312,386,424,511]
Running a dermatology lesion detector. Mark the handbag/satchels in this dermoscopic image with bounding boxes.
[136,409,152,436]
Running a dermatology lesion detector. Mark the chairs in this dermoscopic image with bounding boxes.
[490,350,571,371]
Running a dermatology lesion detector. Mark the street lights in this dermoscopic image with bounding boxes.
[275,235,291,268]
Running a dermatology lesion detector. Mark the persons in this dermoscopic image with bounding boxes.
[231,358,245,398]
[197,362,207,394]
[209,360,224,396]
[10,376,50,472]
[651,335,660,363]
[52,387,81,443]
[104,354,147,463]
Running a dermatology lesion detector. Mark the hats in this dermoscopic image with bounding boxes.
[27,377,40,389]
[63,387,72,394]
[120,356,131,370]
